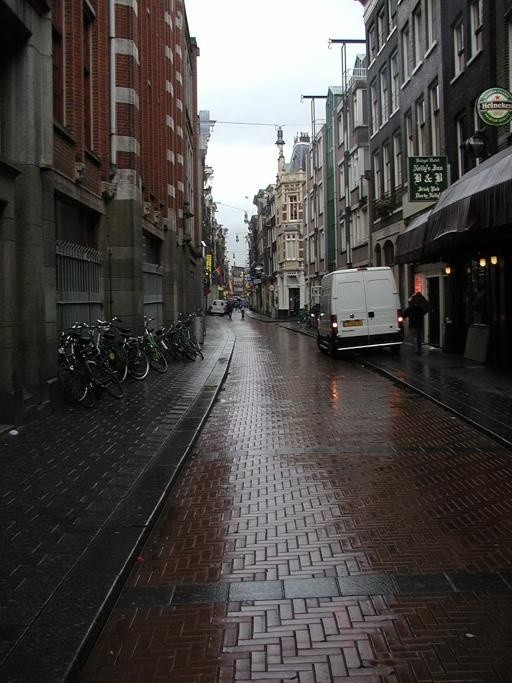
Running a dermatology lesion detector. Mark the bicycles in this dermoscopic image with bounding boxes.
[56,312,204,409]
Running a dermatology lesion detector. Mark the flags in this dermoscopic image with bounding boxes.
[213,267,223,287]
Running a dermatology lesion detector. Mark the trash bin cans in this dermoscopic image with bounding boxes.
[191,317,204,344]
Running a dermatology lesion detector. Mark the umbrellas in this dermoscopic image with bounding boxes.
[239,300,249,307]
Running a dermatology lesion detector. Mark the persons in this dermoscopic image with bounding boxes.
[240,305,246,319]
[223,301,233,320]
[402,300,425,353]
[462,283,477,315]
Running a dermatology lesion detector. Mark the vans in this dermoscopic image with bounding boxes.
[315,267,404,358]
[210,300,225,316]
